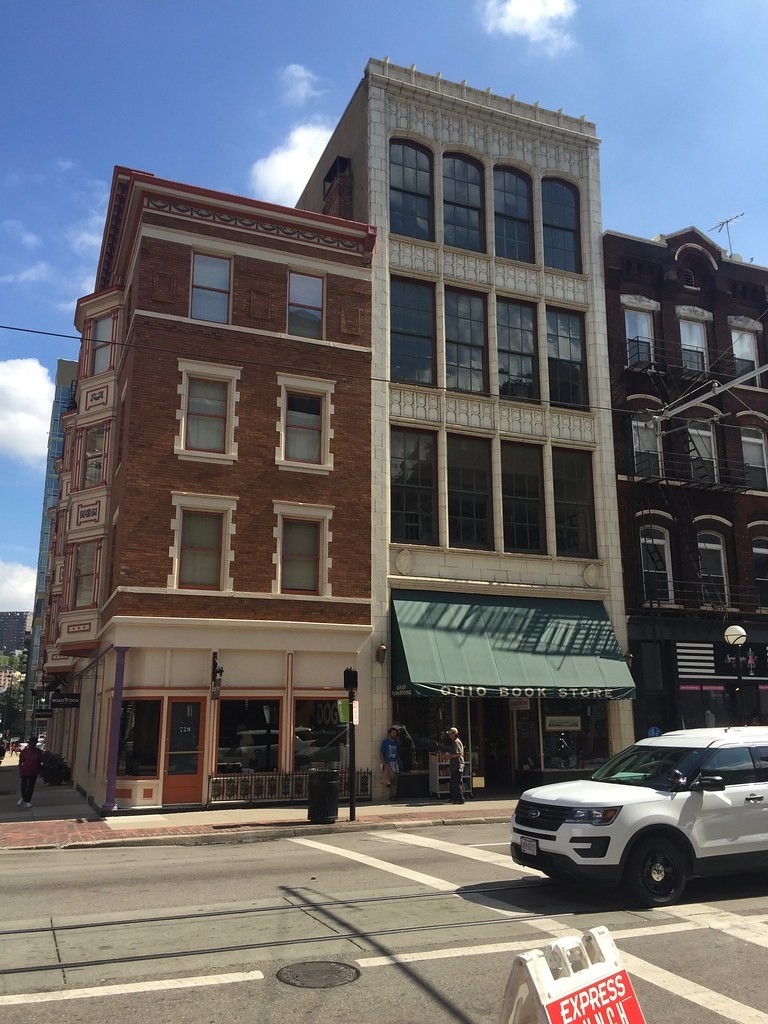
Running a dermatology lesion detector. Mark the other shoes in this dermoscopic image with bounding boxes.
[389,796,399,803]
[24,802,32,808]
[17,797,24,806]
[444,799,453,803]
[453,801,465,804]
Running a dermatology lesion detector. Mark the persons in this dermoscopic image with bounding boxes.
[0,742,5,765]
[444,727,467,805]
[380,727,401,802]
[10,741,18,757]
[17,737,42,807]
[123,729,135,777]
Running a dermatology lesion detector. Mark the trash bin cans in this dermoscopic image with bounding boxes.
[217,763,243,773]
[307,768,341,824]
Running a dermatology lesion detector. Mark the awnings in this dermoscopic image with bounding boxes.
[391,587,636,701]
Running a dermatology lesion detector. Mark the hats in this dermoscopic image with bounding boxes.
[446,727,458,735]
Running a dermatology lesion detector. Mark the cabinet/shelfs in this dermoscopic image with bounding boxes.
[428,751,474,799]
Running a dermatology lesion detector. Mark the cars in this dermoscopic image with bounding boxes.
[212,723,351,769]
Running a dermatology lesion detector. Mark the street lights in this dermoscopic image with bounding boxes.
[725,624,748,725]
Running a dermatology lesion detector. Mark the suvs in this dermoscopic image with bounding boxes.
[508,725,767,909]
